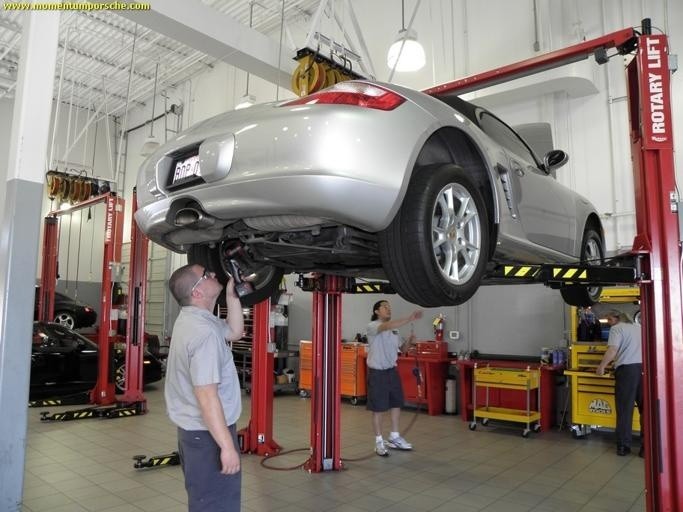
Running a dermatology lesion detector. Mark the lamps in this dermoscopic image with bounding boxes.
[234,1,256,110]
[387,0,426,72]
[140,63,161,156]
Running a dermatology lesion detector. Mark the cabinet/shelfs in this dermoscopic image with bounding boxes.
[564,288,641,437]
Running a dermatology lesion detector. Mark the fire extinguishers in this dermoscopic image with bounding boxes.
[434,313,445,341]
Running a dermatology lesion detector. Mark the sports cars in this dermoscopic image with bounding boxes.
[132,80,604,308]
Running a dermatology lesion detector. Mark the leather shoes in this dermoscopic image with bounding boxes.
[617,445,632,456]
[639,446,644,458]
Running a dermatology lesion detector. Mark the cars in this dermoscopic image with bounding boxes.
[33,321,165,394]
[34,285,97,335]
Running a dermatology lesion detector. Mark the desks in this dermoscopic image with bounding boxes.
[458,360,563,431]
[363,353,449,415]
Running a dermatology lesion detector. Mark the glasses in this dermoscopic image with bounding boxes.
[191,268,211,292]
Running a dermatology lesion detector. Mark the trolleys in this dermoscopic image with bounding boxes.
[468,360,543,438]
[242,344,299,395]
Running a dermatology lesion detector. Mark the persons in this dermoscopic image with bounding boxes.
[596,308,645,457]
[365,299,424,458]
[163,262,245,512]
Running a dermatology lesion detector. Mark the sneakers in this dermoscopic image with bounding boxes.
[374,443,390,458]
[385,436,413,450]
